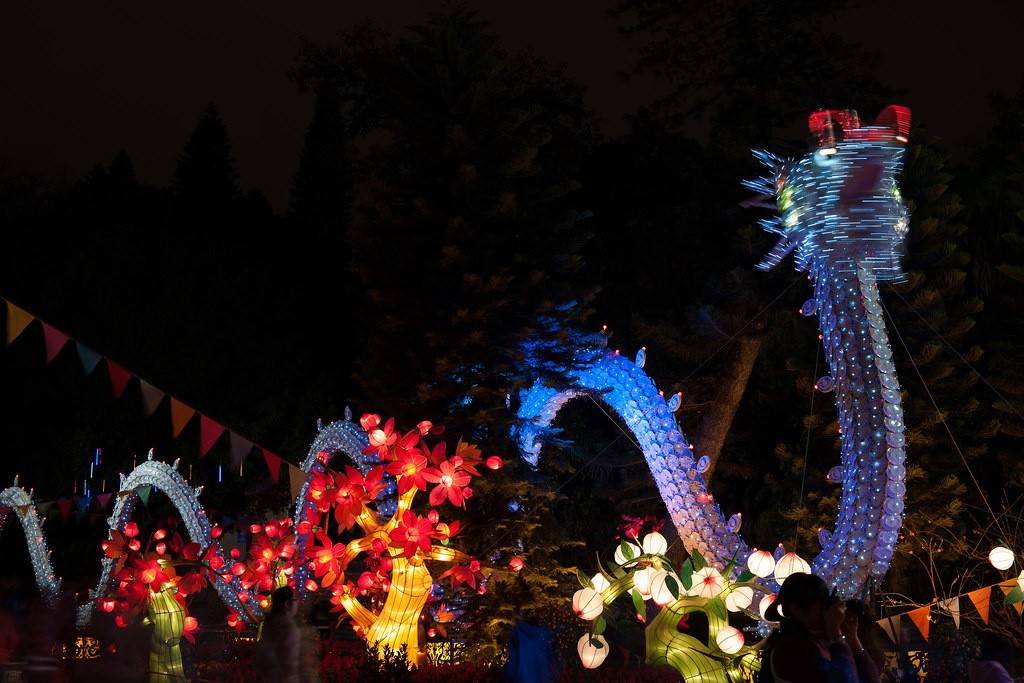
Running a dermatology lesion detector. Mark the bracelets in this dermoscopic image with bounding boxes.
[830,635,847,643]
[853,648,864,654]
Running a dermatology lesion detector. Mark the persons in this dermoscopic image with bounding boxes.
[766,573,1024,683]
[259,587,301,683]
[505,620,550,683]
[0,607,19,683]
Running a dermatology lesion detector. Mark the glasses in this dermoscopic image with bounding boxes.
[290,597,299,603]
